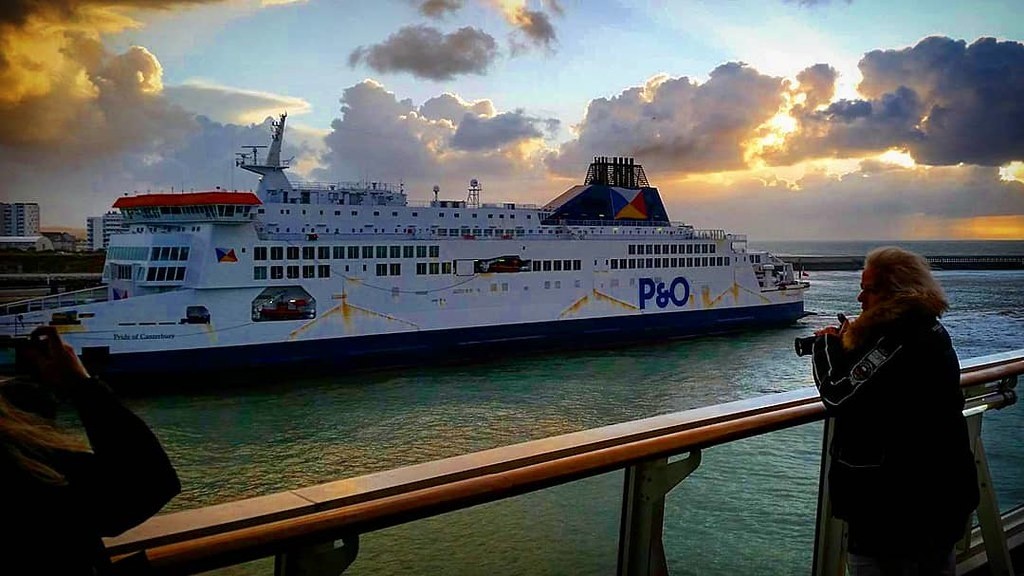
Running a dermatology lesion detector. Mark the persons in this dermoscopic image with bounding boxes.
[811,246,979,576]
[0,325,182,576]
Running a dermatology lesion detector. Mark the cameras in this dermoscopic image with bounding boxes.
[794,314,848,357]
[0,334,40,377]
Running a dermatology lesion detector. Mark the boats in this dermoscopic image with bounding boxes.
[476,257,521,272]
[1,111,818,402]
[261,306,310,319]
